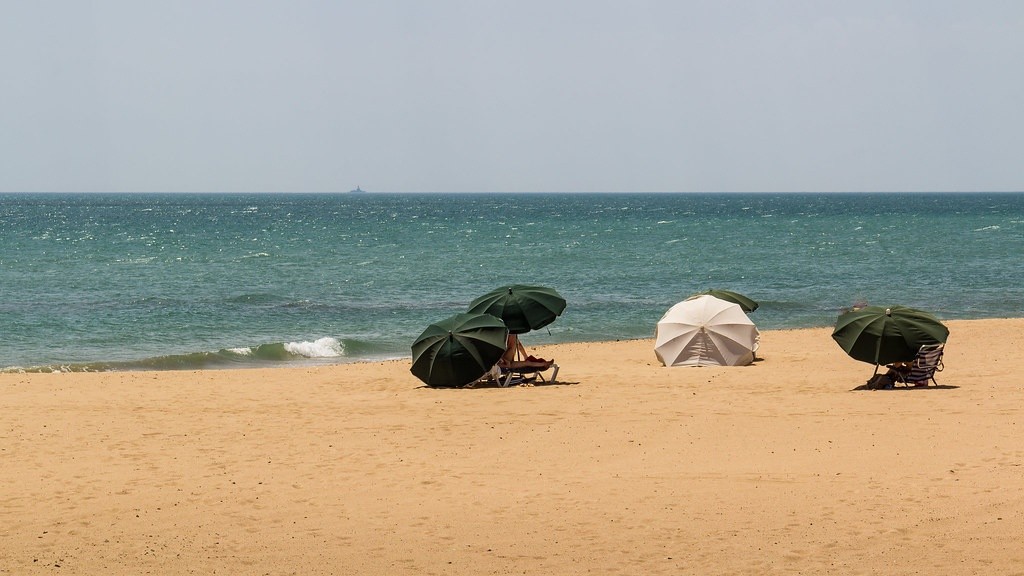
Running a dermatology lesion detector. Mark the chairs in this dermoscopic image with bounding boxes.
[490,356,559,388]
[885,344,946,388]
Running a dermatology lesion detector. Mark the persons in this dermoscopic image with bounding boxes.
[497,334,554,369]
[893,361,914,366]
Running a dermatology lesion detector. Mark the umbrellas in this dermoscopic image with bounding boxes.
[833,303,950,366]
[468,284,567,334]
[410,314,507,389]
[655,288,761,367]
